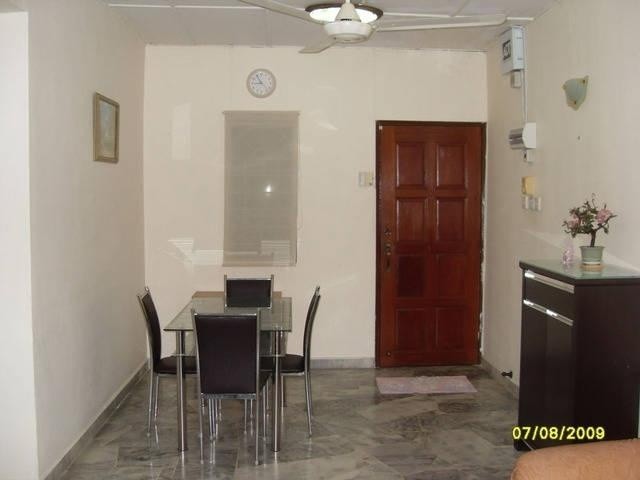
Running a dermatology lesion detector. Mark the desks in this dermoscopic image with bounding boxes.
[164,296,292,451]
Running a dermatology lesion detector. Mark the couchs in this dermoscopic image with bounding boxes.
[510,439,640,480]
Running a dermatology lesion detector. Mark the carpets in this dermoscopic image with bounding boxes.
[376,375,477,395]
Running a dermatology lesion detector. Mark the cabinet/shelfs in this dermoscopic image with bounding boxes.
[515,259,640,451]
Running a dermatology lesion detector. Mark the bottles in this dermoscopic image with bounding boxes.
[562,235,574,265]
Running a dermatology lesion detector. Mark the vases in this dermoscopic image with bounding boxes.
[580,246,606,265]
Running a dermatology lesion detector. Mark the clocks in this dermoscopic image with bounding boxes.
[246,69,276,98]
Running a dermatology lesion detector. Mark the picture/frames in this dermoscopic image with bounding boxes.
[94,92,120,163]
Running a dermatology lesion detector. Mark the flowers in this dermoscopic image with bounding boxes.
[562,198,617,246]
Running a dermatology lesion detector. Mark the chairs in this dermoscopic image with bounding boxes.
[223,274,274,354]
[137,287,196,437]
[260,286,322,435]
[190,309,272,465]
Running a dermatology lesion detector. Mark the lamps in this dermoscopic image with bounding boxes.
[304,3,384,23]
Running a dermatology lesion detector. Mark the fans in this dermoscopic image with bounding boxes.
[239,0,507,54]
